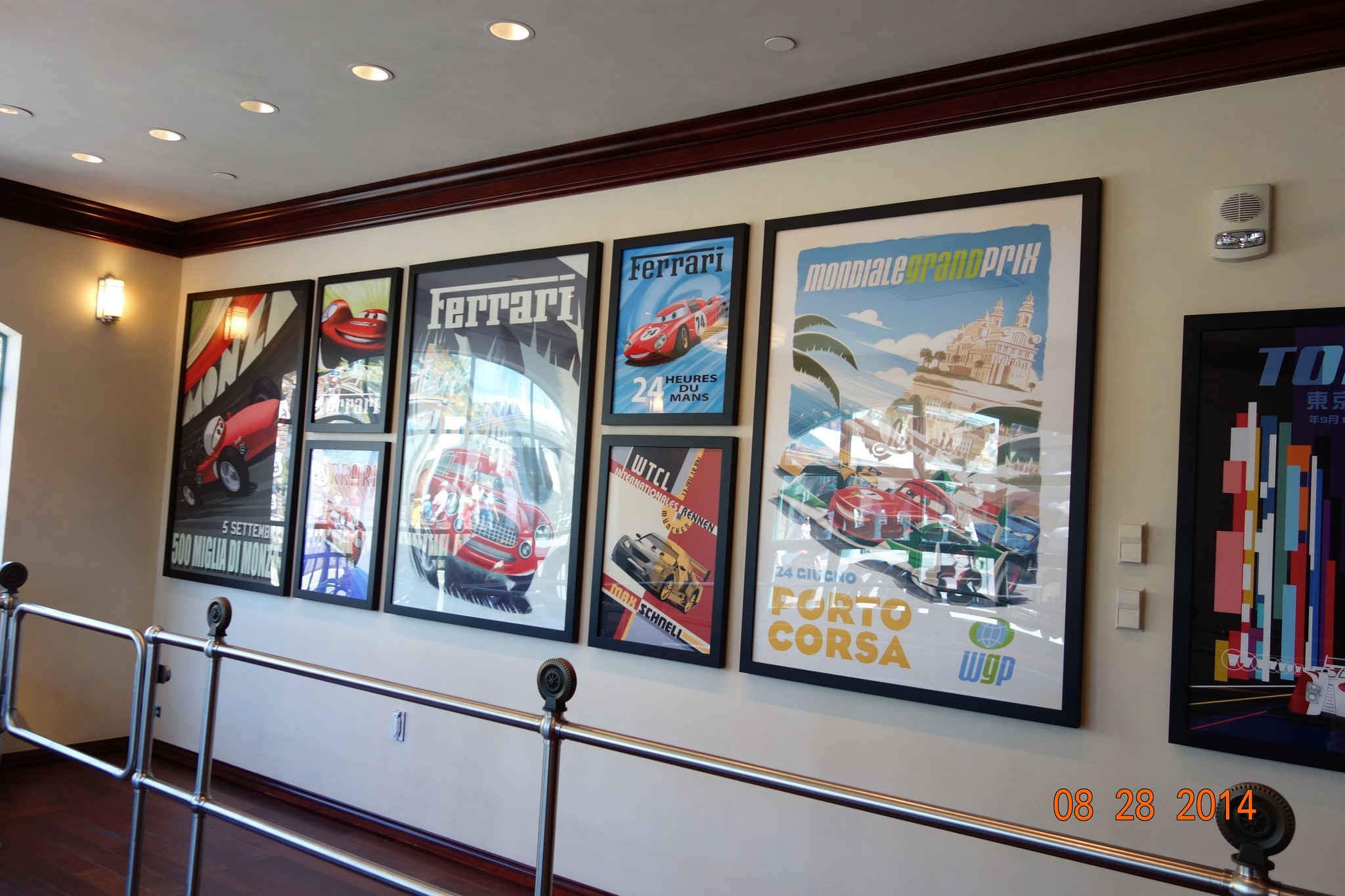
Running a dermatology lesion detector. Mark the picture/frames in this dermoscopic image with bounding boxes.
[163,178,1104,731]
[1168,307,1345,773]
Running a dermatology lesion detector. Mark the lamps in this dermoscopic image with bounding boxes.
[96,274,125,325]
